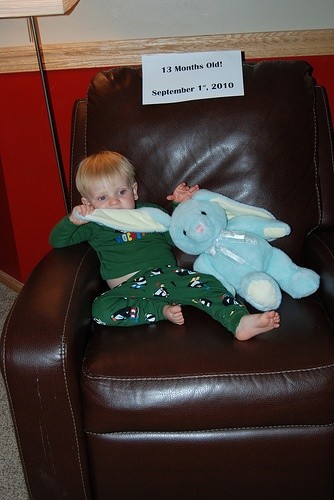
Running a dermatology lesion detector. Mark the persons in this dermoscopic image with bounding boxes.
[48,149,281,341]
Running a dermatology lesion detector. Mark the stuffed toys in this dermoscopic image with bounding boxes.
[74,188,320,311]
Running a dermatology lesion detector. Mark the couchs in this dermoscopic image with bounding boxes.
[0,58,334,500]
